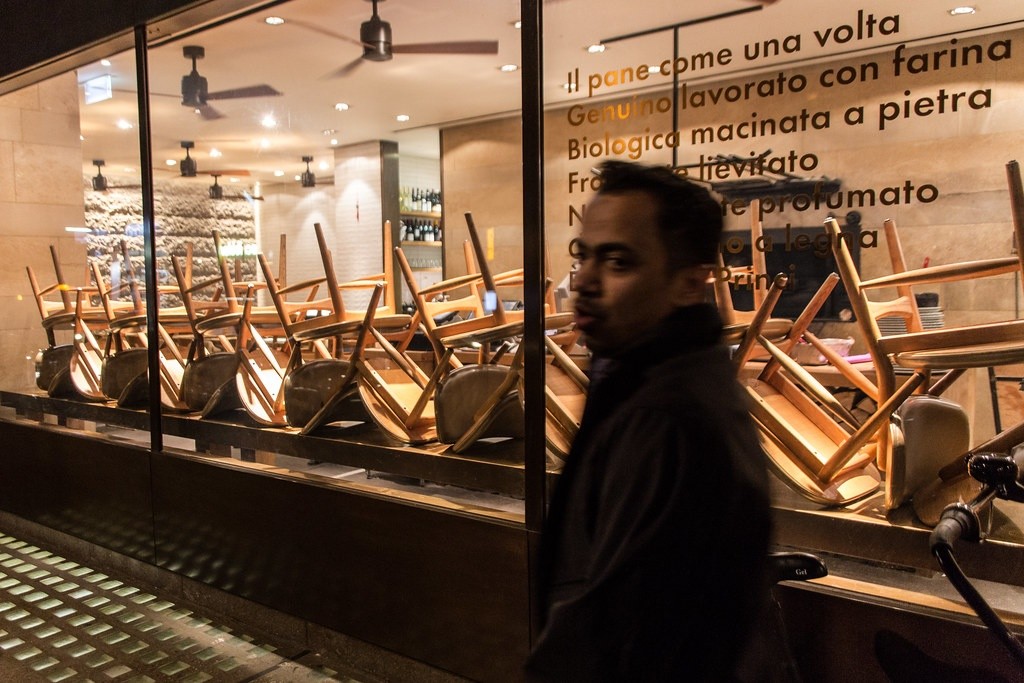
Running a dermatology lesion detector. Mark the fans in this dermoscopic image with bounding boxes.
[155,141,247,177]
[286,0,498,80]
[91,158,139,190]
[94,46,279,122]
[277,154,333,187]
[201,175,261,201]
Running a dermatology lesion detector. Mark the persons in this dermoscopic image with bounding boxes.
[518,159,775,683]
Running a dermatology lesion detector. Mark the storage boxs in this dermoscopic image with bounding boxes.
[796,338,854,366]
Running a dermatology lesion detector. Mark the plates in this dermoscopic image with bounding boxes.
[876,305,949,374]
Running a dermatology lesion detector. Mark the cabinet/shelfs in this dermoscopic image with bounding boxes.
[398,207,443,271]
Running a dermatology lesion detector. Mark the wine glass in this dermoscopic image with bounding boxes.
[407,253,439,268]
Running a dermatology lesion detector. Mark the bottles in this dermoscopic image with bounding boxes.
[400,187,411,211]
[430,190,441,212]
[428,220,434,242]
[414,219,420,241]
[419,220,424,241]
[411,301,416,317]
[403,219,414,241]
[424,220,429,242]
[442,292,448,302]
[416,188,422,211]
[421,190,426,212]
[434,220,442,241]
[425,189,432,212]
[411,188,417,211]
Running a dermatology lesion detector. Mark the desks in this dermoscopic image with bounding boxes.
[740,361,939,395]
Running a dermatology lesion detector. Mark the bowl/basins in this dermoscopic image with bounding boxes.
[915,294,939,308]
[797,338,855,364]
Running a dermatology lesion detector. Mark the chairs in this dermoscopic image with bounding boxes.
[24,154,1023,540]
